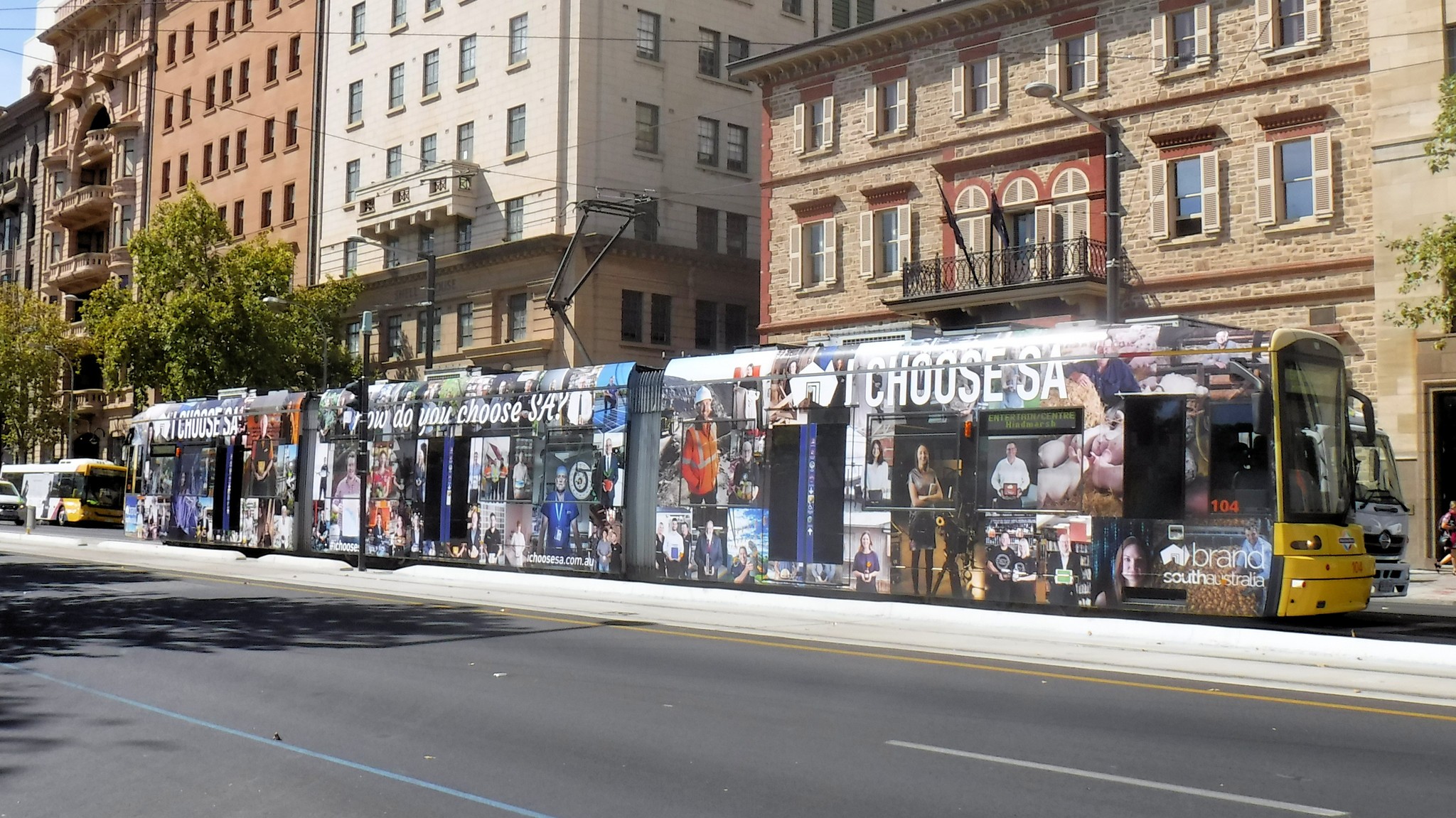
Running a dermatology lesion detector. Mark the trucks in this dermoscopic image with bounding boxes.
[1345,413,1412,599]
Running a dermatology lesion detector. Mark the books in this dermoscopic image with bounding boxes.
[929,483,937,495]
[1040,538,1092,605]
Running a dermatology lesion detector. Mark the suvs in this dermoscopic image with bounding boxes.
[0,480,26,526]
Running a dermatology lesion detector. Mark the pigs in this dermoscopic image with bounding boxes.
[1038,421,1124,508]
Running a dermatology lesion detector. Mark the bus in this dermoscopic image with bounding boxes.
[0,457,126,527]
[126,315,1378,623]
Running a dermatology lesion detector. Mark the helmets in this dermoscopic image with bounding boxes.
[694,386,712,406]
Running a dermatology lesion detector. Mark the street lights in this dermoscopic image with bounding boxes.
[261,296,328,390]
[347,235,435,369]
[44,345,75,459]
[1025,79,1122,316]
[357,311,373,572]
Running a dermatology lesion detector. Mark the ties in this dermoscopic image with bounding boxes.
[707,539,709,547]
[607,458,609,473]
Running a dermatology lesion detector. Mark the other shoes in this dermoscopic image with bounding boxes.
[1434,561,1441,573]
[1452,570,1456,575]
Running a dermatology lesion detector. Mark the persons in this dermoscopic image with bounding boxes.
[906,442,943,596]
[1001,347,1026,409]
[1094,536,1153,607]
[1434,501,1456,575]
[1063,336,1141,428]
[991,442,1030,509]
[656,361,800,585]
[986,531,1037,603]
[852,531,880,591]
[835,359,846,383]
[132,376,622,575]
[811,563,836,582]
[860,440,888,502]
[1230,523,1272,586]
[1202,330,1239,369]
[872,366,883,399]
[1046,533,1083,606]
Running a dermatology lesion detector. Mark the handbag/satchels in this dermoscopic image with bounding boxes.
[1439,536,1453,548]
[1438,512,1452,531]
